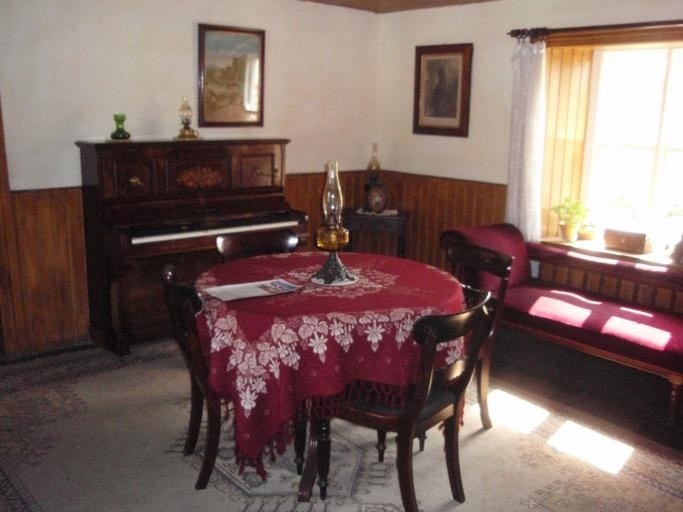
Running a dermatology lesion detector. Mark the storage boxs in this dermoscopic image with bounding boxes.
[603,225,654,255]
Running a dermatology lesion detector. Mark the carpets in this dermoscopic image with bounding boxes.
[2,336,683,510]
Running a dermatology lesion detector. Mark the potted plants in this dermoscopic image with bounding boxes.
[554,196,586,240]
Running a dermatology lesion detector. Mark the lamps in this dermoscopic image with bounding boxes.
[173,96,201,141]
[309,163,358,287]
[366,140,382,185]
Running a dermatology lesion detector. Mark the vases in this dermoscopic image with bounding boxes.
[110,114,130,139]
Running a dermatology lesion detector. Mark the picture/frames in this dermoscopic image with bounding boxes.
[411,44,473,138]
[197,24,265,129]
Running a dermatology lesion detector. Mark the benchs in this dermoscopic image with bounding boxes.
[439,220,682,430]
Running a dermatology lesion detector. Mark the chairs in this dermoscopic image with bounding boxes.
[314,289,496,510]
[159,258,306,491]
[411,228,513,454]
[216,227,302,259]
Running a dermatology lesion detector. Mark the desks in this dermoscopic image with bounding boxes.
[341,210,413,259]
[194,247,469,477]
